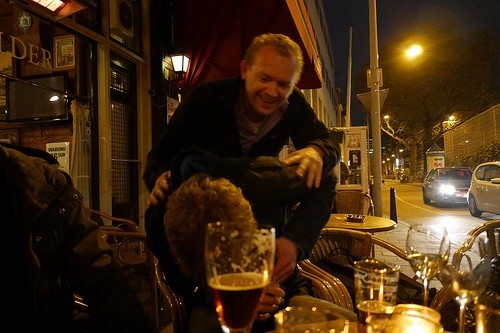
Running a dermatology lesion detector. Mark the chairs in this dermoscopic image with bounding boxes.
[298,188,500,333]
[78,203,174,315]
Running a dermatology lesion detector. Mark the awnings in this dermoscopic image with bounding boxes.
[168,0,323,99]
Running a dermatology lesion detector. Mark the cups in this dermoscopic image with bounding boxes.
[353,259,443,333]
[275,306,350,333]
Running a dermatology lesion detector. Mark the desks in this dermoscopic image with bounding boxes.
[325,213,396,259]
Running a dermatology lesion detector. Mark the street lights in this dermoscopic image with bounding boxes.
[381,148,406,179]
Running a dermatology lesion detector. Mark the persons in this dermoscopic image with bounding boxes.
[137,34,341,204]
[142,148,340,333]
[340,160,349,185]
[1,140,178,333]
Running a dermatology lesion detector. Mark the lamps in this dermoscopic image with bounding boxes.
[168,47,190,81]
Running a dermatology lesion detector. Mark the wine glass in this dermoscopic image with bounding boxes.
[206,221,275,333]
[437,235,491,333]
[405,222,451,306]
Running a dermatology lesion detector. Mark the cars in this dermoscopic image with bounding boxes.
[421,167,475,207]
[467,161,500,218]
